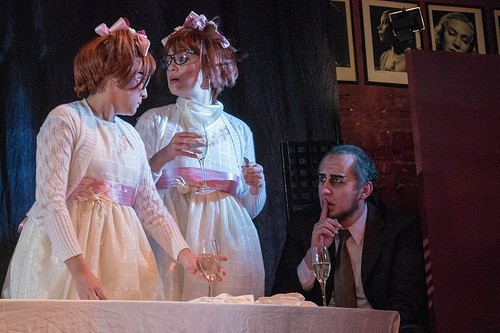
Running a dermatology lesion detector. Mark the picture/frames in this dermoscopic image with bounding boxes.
[360,0,424,88]
[329,0,359,84]
[426,2,488,55]
[490,8,500,55]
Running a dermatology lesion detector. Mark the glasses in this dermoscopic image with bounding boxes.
[137,71,151,90]
[159,49,200,69]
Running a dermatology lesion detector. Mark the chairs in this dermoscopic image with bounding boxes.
[281,139,346,225]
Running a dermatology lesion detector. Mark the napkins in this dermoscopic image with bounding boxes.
[193,291,257,305]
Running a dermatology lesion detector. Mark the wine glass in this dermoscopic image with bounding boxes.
[311,246,331,307]
[199,238,219,297]
[189,124,217,194]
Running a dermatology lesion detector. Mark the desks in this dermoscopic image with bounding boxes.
[0,299,400,333]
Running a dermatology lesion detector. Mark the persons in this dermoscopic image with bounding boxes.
[377,10,477,72]
[138,11,267,300]
[276,146,425,333]
[1,17,229,302]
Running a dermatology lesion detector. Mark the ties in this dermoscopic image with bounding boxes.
[333,229,357,309]
